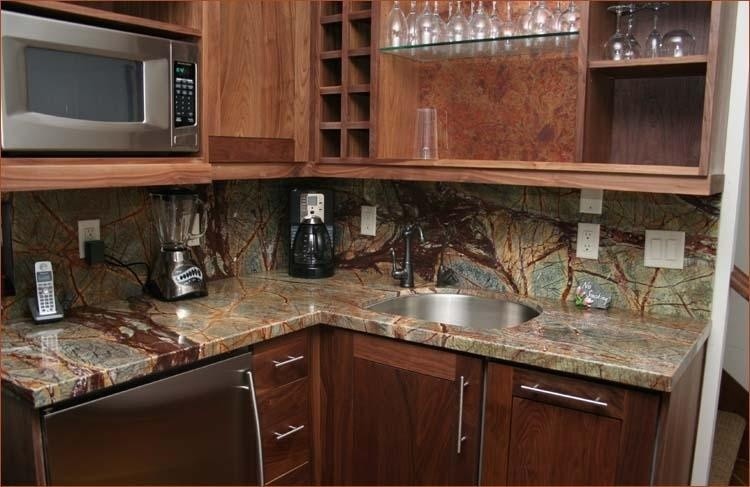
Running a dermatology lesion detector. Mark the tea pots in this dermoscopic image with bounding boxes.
[290,216,335,278]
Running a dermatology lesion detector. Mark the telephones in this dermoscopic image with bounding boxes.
[28,261,64,324]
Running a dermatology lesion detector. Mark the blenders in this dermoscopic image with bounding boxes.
[144,186,211,300]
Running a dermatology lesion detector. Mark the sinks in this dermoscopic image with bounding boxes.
[355,286,545,332]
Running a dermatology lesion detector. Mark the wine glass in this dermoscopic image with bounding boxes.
[384,0,584,47]
[601,2,670,61]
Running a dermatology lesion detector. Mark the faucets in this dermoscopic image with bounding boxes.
[388,223,444,291]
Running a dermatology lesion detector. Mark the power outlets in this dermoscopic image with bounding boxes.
[360,205,377,236]
[77,218,101,259]
[575,222,600,260]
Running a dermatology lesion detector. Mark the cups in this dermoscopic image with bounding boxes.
[412,106,439,161]
[662,28,696,59]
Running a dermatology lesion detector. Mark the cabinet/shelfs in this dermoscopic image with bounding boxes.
[480,344,705,487]
[253,338,317,485]
[375,2,583,175]
[317,325,482,487]
[580,2,713,179]
[313,1,377,167]
[206,2,310,167]
[1,1,210,193]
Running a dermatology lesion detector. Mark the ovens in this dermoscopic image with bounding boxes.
[40,349,266,486]
[0,9,203,159]
